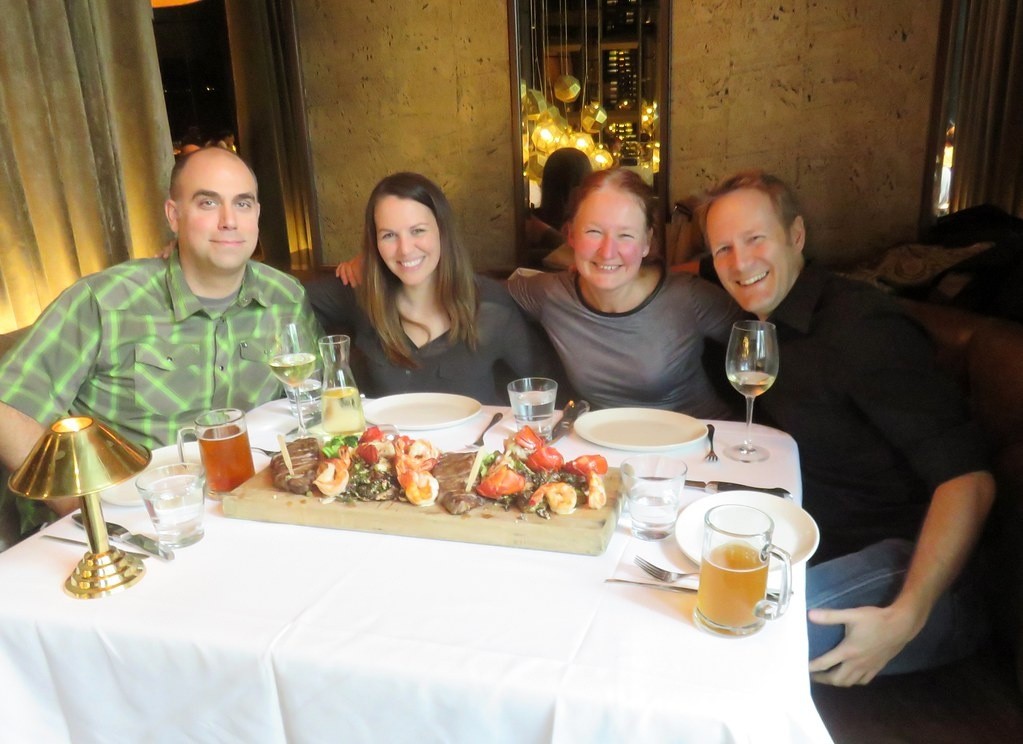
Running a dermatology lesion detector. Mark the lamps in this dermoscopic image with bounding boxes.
[5,414,153,593]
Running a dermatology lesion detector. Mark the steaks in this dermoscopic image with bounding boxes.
[268,437,319,494]
[429,449,481,515]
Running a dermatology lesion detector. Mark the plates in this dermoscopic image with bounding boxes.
[98,442,198,506]
[674,491,820,569]
[573,407,709,450]
[363,392,485,430]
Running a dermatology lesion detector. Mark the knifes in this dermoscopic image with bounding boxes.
[285,414,323,436]
[42,512,175,561]
[641,475,790,500]
[541,399,590,444]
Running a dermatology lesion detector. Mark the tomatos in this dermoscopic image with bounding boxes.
[359,426,439,474]
[475,426,609,498]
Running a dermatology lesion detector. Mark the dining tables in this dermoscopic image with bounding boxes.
[0,398,837,744]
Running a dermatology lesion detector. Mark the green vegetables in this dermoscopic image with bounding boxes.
[479,449,589,519]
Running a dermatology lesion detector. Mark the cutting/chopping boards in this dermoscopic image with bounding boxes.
[220,453,624,555]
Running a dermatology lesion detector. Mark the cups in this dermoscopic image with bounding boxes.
[283,369,322,429]
[507,377,558,440]
[692,504,791,636]
[620,455,687,540]
[177,408,255,500]
[135,462,204,549]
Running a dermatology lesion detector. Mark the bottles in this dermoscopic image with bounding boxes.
[317,335,367,436]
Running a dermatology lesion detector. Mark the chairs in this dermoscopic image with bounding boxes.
[875,299,1023,632]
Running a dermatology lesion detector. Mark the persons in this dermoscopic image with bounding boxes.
[1,145,321,538]
[521,148,592,261]
[335,167,759,422]
[695,169,1021,690]
[305,170,574,411]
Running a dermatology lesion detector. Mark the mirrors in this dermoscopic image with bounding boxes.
[920,0,1022,227]
[505,0,670,272]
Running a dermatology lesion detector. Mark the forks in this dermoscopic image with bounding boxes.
[635,555,796,598]
[703,424,718,461]
[465,412,503,446]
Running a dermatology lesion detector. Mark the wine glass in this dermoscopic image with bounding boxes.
[725,320,779,462]
[265,316,323,446]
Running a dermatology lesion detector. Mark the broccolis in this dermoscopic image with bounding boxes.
[320,435,359,460]
